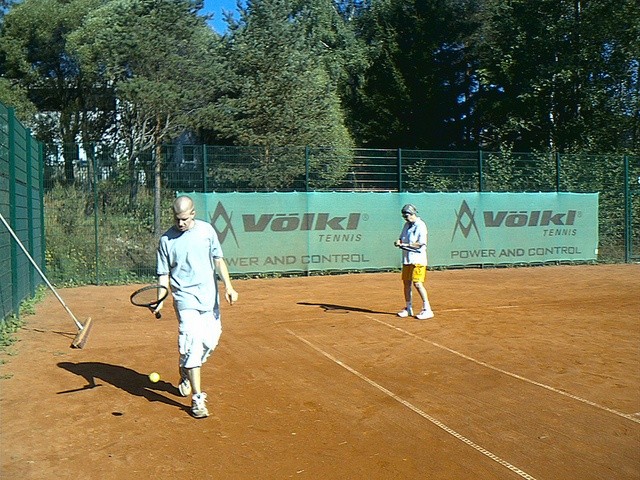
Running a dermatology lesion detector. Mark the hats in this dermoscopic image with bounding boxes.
[403,205,417,213]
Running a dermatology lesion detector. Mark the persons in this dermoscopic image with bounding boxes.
[393,203,437,321]
[149,194,241,420]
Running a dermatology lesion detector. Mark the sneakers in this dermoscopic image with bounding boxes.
[398,307,414,317]
[416,309,434,319]
[191,392,210,418]
[179,368,192,396]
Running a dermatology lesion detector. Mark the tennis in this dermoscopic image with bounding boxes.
[148,372,160,383]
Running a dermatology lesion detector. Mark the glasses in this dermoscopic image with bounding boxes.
[402,214,410,218]
